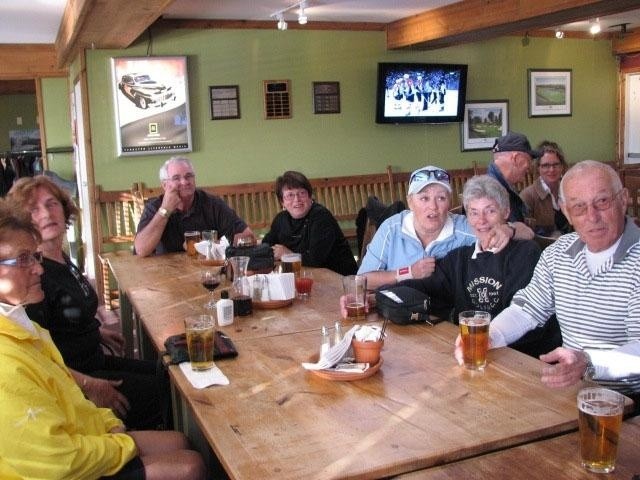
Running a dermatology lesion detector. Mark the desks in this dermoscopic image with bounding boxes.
[125,268,640,480]
[106,245,414,439]
[370,418,639,478]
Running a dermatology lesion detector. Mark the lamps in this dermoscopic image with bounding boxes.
[587,17,601,35]
[554,24,564,39]
[269,1,309,30]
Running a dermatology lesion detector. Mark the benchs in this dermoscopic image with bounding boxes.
[93,160,621,311]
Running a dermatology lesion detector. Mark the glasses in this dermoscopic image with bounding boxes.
[283,189,308,200]
[409,169,451,185]
[566,189,622,216]
[539,162,561,169]
[0,251,43,267]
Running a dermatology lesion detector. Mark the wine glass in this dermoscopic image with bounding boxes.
[199,266,221,310]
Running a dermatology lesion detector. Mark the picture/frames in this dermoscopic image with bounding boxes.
[527,68,573,118]
[460,99,509,153]
[110,54,193,157]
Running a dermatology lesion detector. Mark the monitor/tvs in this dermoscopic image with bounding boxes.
[376,62,468,123]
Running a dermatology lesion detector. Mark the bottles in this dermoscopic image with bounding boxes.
[230,255,253,317]
[253,275,261,300]
[217,290,234,327]
[333,321,343,348]
[319,327,331,362]
[261,275,272,303]
[205,242,214,261]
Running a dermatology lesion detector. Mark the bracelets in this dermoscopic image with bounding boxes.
[80,374,90,392]
[504,221,517,240]
[396,267,415,284]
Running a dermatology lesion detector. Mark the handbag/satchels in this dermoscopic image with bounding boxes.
[224,243,275,272]
[374,286,449,327]
[155,330,238,376]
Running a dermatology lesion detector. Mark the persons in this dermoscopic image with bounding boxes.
[259,172,360,276]
[518,139,577,239]
[355,164,537,305]
[385,69,460,116]
[487,132,556,253]
[454,160,640,424]
[39,170,77,261]
[340,176,561,365]
[1,198,209,480]
[133,155,256,256]
[6,176,166,428]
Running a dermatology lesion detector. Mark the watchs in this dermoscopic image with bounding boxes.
[157,207,168,219]
[578,350,596,383]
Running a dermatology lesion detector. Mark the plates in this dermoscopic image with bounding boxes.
[253,297,294,309]
[306,350,384,381]
[246,265,277,276]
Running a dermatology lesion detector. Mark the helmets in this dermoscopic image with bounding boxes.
[403,74,409,79]
[396,80,401,84]
[417,76,422,80]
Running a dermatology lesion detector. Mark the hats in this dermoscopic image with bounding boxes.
[491,133,538,157]
[407,165,452,196]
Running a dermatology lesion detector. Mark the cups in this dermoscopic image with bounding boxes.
[295,270,314,300]
[281,253,301,279]
[458,309,491,370]
[343,274,368,322]
[185,231,202,259]
[576,387,625,474]
[235,235,254,247]
[524,219,537,231]
[184,315,216,372]
[350,336,385,367]
[202,229,219,242]
